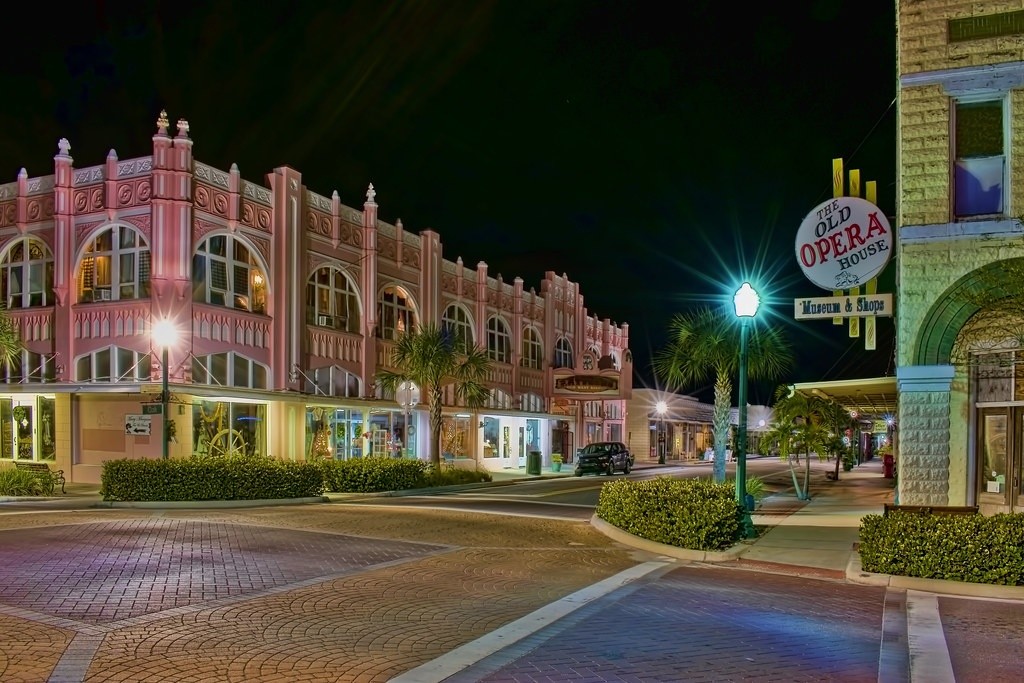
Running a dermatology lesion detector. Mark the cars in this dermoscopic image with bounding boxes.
[575,442,635,477]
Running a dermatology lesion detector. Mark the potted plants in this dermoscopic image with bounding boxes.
[552,453,562,472]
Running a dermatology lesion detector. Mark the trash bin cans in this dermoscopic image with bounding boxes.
[526,451,542,475]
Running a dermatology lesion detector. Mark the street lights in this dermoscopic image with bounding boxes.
[733,282,760,537]
[157,318,174,459]
[656,402,667,464]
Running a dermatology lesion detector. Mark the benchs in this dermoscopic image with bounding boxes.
[12,461,66,494]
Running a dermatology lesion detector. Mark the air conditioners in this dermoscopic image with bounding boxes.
[319,316,335,329]
[92,289,111,302]
